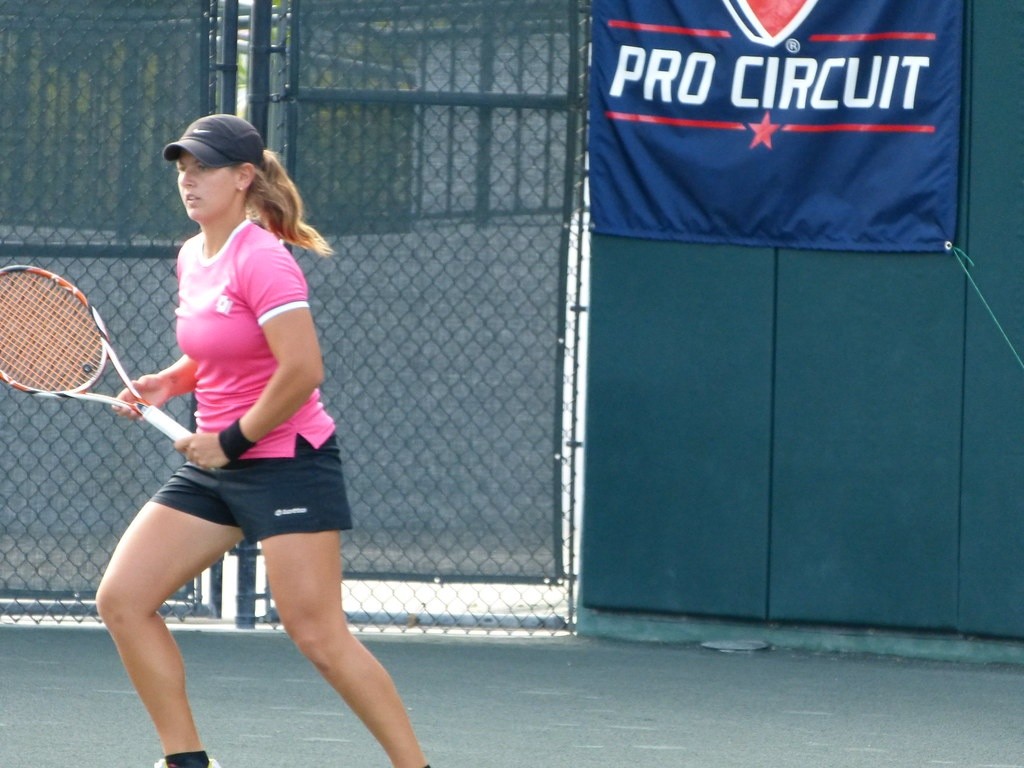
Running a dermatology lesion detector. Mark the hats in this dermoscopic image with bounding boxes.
[164,114,265,169]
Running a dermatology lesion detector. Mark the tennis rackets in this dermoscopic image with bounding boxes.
[0,265,210,466]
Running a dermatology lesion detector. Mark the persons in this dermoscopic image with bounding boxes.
[94,113,427,768]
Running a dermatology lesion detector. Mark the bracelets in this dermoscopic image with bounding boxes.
[218,417,257,463]
